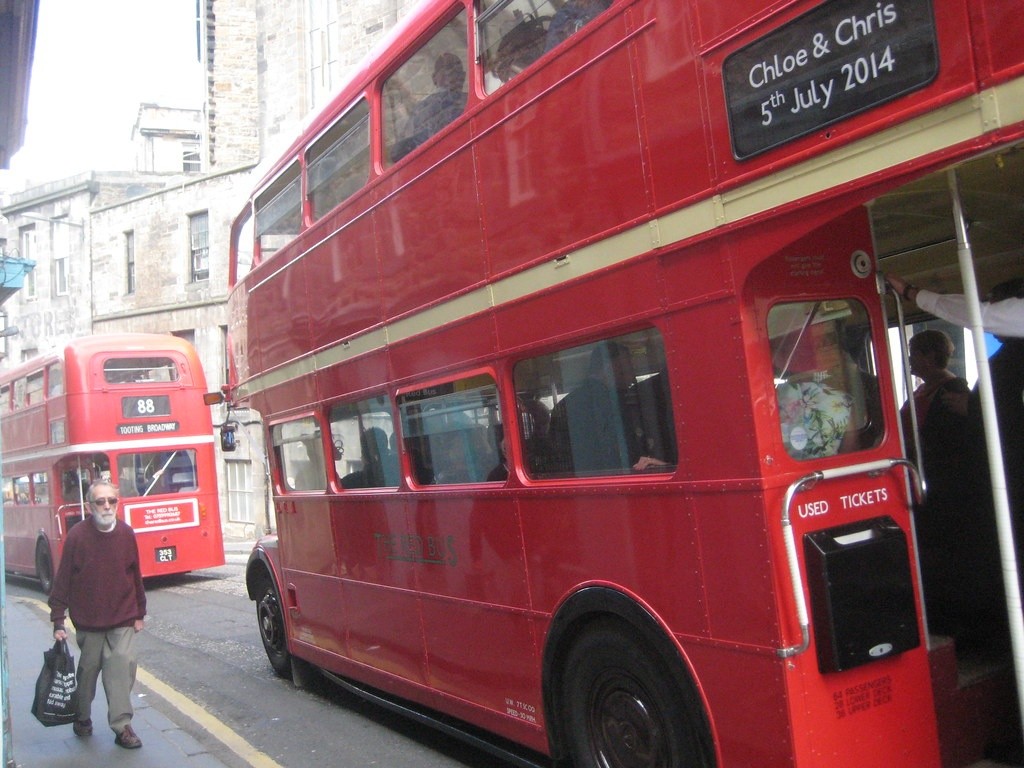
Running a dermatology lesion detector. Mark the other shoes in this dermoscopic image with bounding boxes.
[73,718,93,737]
[114,724,142,748]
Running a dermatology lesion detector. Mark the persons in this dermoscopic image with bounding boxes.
[48,481,146,748]
[397,52,468,160]
[64,466,163,501]
[544,0,614,55]
[774,270,1024,767]
[296,340,677,491]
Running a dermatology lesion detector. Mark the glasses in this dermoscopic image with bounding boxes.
[90,497,117,505]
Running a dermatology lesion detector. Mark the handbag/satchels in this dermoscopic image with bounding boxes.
[30,638,79,728]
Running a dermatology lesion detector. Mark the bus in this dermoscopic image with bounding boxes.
[203,0,1024,767]
[1,332,226,595]
